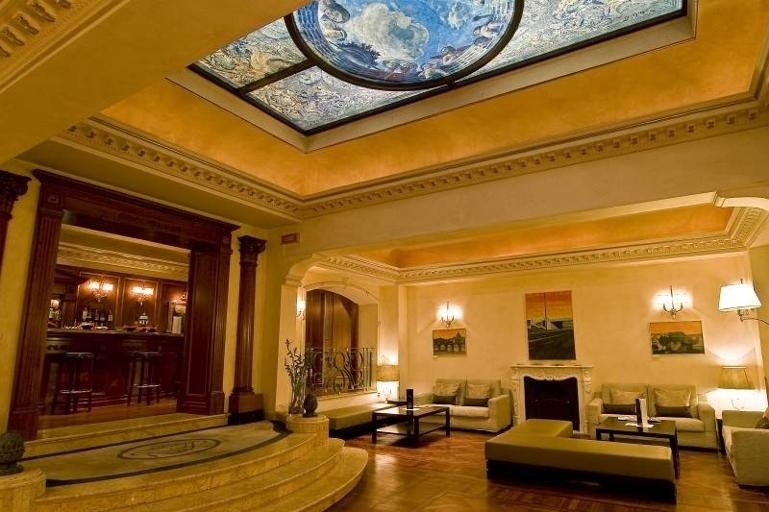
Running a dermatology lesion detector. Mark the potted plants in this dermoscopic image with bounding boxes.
[283,338,317,414]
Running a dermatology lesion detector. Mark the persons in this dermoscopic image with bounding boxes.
[318,0,504,82]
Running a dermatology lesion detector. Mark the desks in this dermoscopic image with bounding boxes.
[385,398,408,423]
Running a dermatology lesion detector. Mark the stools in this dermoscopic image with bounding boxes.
[126,351,160,406]
[50,352,95,415]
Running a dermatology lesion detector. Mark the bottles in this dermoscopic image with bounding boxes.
[82,306,114,322]
[406,388,413,409]
[49,303,61,322]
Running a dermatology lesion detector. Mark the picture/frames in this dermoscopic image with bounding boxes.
[432,327,467,359]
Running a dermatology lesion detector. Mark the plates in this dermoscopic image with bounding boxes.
[96,326,107,331]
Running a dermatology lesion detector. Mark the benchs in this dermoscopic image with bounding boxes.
[316,402,394,439]
[484,419,676,503]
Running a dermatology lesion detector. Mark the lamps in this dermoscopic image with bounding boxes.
[718,366,757,409]
[718,282,769,326]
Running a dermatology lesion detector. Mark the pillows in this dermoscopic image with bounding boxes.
[462,397,489,408]
[654,387,692,407]
[755,407,769,428]
[431,392,456,405]
[610,387,640,405]
[433,382,461,404]
[655,404,693,418]
[467,384,491,398]
[603,403,636,415]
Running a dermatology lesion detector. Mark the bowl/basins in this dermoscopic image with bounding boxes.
[80,325,94,330]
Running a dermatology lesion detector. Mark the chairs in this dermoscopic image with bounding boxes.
[720,408,769,488]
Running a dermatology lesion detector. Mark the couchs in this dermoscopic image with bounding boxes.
[586,382,720,455]
[408,378,512,434]
